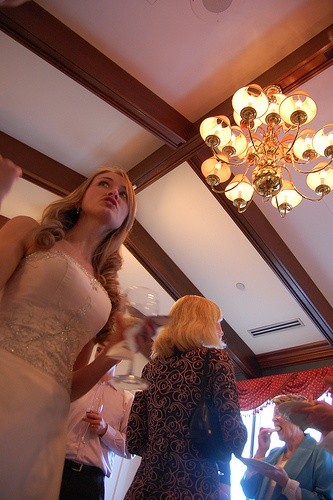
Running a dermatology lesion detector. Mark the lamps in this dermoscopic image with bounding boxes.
[200,83,333,218]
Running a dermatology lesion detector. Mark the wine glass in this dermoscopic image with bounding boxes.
[72,399,104,449]
[109,288,159,392]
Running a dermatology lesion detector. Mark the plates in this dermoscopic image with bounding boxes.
[241,458,279,470]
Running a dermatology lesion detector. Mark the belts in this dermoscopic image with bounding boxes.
[63,458,105,478]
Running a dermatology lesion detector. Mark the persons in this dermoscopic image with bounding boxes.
[0,155,158,500]
[125,295,247,500]
[238,394,333,500]
[56,335,137,500]
[291,372,333,455]
